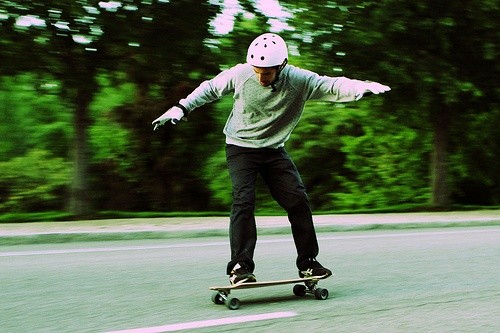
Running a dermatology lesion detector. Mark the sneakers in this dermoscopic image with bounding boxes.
[229,262,256,286]
[299,258,332,279]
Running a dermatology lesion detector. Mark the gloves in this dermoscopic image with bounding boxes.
[355,81,391,101]
[152,105,188,131]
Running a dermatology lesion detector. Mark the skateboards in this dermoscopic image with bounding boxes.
[209,273,329,310]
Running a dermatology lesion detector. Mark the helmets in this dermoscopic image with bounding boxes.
[246,33,288,68]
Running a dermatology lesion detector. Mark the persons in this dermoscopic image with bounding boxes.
[152,34,392,282]
